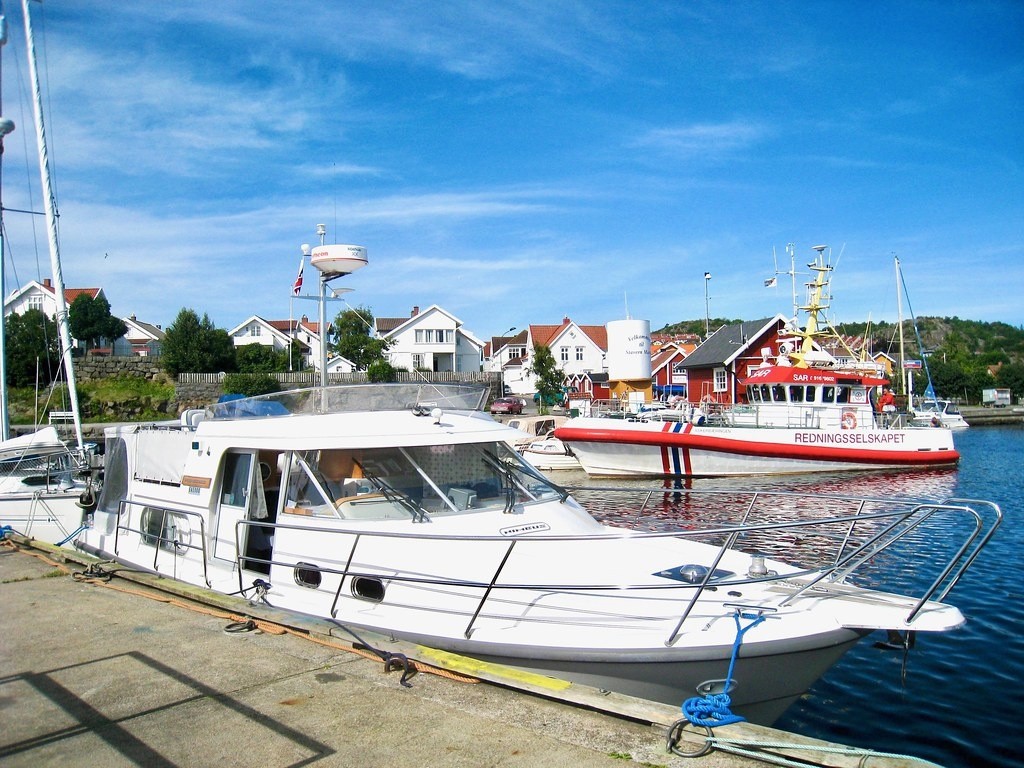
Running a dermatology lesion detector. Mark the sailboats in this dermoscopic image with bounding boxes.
[0,0,1002,707]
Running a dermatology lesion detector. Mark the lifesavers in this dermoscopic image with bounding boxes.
[841,412,857,429]
[699,394,717,411]
[672,397,685,409]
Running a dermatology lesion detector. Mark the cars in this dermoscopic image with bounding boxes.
[490,396,526,415]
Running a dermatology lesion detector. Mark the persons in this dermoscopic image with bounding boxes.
[930,416,944,428]
[874,387,895,414]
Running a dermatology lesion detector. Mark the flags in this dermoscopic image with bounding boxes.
[765,277,776,287]
[293,256,305,296]
